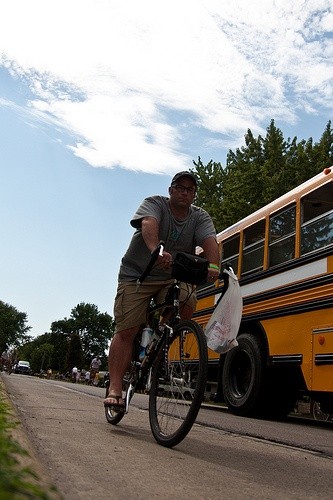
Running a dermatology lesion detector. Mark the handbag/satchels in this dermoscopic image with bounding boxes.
[204,269,242,354]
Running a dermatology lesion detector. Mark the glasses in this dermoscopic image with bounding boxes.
[172,185,195,193]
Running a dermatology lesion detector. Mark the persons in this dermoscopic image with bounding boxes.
[39,355,109,388]
[104,171,219,407]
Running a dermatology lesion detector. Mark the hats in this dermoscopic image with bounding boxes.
[172,171,196,184]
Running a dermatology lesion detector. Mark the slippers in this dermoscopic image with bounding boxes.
[143,374,171,385]
[104,395,125,407]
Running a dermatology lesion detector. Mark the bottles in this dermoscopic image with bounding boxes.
[139,325,154,361]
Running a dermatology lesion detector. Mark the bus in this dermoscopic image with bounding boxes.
[167,166,333,424]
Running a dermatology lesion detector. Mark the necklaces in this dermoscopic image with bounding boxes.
[170,208,187,219]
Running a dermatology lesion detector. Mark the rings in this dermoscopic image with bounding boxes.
[210,276,215,279]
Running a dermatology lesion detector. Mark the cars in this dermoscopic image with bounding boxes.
[12,361,31,375]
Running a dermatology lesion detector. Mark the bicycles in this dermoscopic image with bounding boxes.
[87,369,99,386]
[103,239,233,449]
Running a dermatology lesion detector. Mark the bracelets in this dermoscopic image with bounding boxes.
[209,264,220,270]
